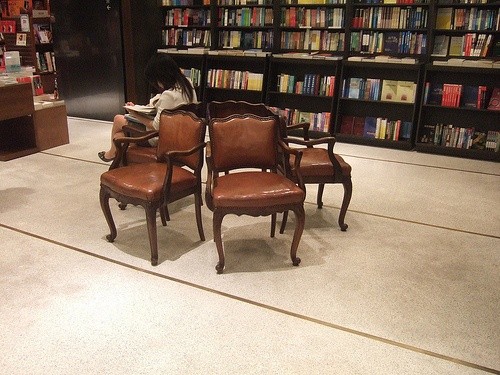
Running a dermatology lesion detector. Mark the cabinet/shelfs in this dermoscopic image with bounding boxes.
[0,0,500,163]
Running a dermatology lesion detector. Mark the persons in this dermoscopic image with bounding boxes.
[98,53,198,163]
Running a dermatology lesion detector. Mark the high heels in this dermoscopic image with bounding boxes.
[98,151,115,162]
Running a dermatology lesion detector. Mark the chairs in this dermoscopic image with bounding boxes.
[99,100,353,272]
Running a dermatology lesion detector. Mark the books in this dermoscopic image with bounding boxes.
[36,52,56,72]
[277,73,335,96]
[163,0,274,5]
[4,51,21,73]
[166,8,274,26]
[435,8,500,30]
[267,106,330,133]
[419,123,500,151]
[280,0,496,4]
[352,7,427,28]
[281,29,495,57]
[478,86,500,110]
[424,82,462,107]
[343,78,417,103]
[280,6,345,28]
[340,115,411,141]
[161,28,273,49]
[16,33,27,45]
[30,75,43,96]
[207,69,263,90]
[433,59,500,69]
[180,68,200,86]
[33,23,53,43]
[124,104,156,115]
